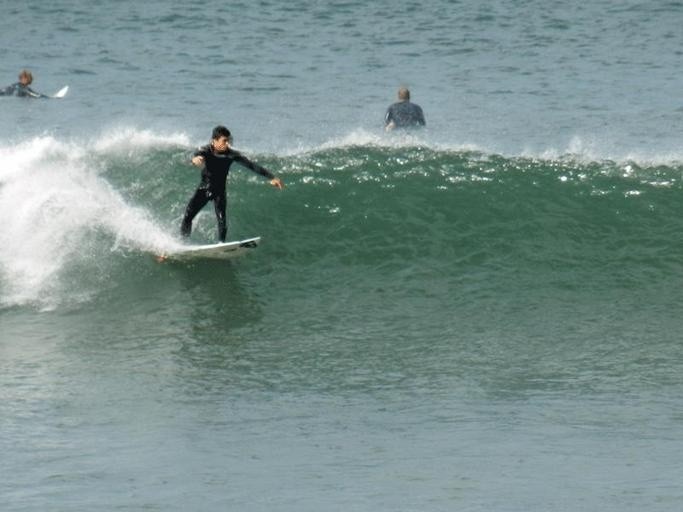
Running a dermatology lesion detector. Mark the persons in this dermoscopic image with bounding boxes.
[181,125,283,243]
[385,88,427,130]
[0,69,62,99]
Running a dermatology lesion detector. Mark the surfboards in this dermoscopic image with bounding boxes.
[186,236,261,259]
[51,85,69,97]
